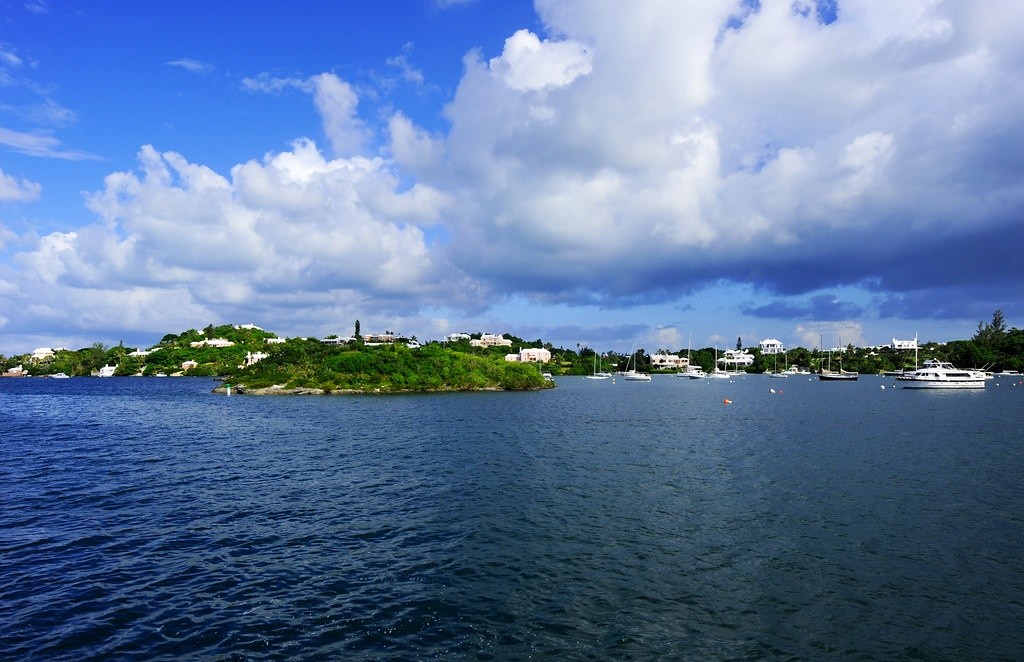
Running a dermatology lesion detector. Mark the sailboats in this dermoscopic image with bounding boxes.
[729,355,747,375]
[706,343,731,379]
[768,345,787,378]
[587,348,608,380]
[622,341,652,383]
[676,332,705,380]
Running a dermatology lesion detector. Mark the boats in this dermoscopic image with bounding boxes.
[783,364,812,375]
[990,369,1024,377]
[817,336,859,381]
[897,331,986,389]
